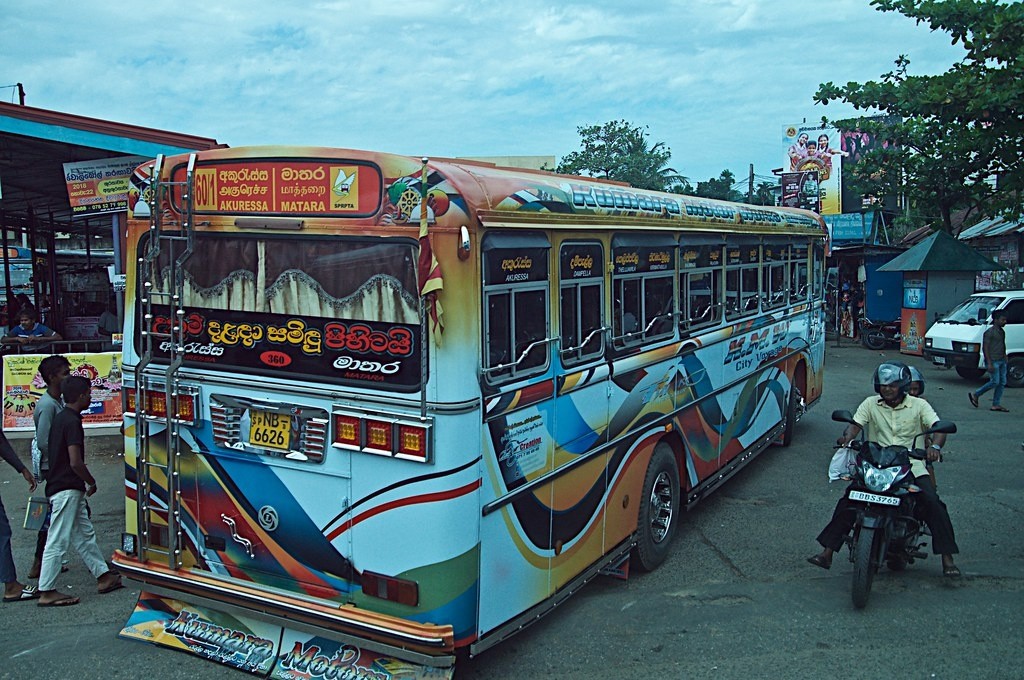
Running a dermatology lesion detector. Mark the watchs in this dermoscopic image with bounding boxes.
[930,444,941,451]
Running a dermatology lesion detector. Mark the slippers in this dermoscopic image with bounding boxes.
[990,407,1009,412]
[3,584,41,602]
[37,595,78,607]
[942,565,961,577]
[968,392,978,408]
[806,554,831,569]
[28,566,68,579]
[98,574,124,594]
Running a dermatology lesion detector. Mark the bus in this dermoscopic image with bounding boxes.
[105,144,829,680]
[0,244,115,340]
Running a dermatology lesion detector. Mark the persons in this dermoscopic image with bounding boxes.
[0,293,62,353]
[0,427,38,603]
[807,360,962,578]
[968,309,1010,412]
[28,355,126,607]
[98,295,121,351]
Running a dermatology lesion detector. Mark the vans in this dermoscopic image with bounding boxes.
[922,288,1024,390]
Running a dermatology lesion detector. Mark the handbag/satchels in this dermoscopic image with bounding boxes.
[828,445,857,483]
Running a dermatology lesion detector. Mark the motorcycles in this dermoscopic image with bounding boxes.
[859,311,904,350]
[831,409,957,609]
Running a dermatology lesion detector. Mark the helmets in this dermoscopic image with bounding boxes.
[873,361,912,394]
[909,365,925,395]
